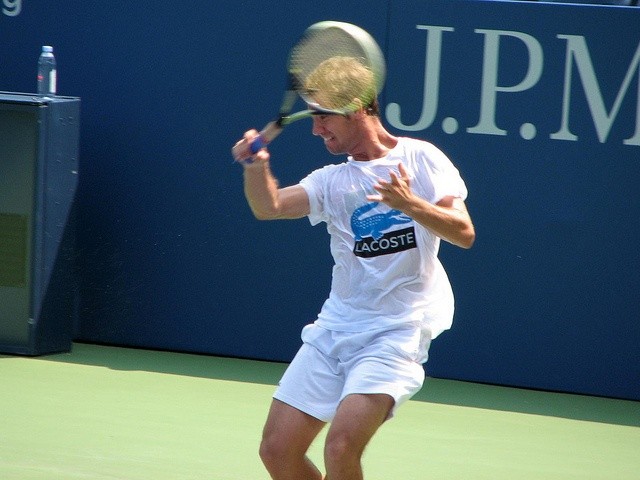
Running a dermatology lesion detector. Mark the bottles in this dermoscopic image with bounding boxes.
[38,46,57,97]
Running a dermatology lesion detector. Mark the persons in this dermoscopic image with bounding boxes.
[233,56,475,479]
